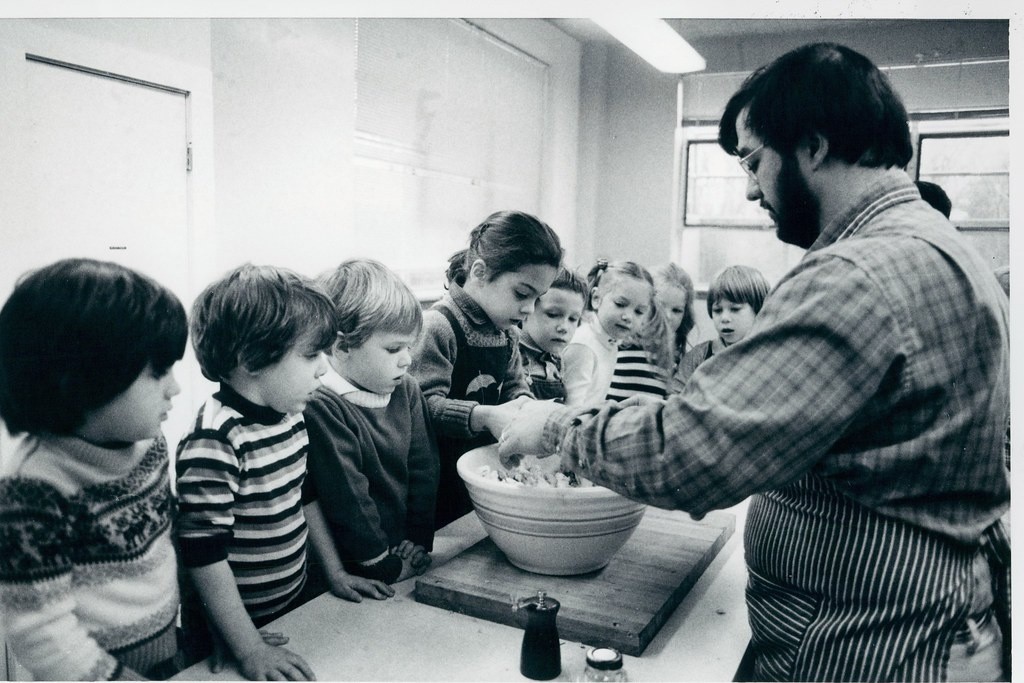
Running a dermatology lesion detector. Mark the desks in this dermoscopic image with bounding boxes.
[589,18,707,74]
[169,489,755,682]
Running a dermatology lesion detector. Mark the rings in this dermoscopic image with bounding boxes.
[502,433,505,442]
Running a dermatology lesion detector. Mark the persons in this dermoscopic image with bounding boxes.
[608,265,695,404]
[508,269,588,406]
[676,264,771,400]
[406,208,563,524]
[0,259,290,681]
[562,259,674,409]
[302,259,438,585]
[176,263,394,682]
[496,41,1010,683]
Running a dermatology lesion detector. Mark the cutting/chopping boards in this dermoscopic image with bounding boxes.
[416,507,736,658]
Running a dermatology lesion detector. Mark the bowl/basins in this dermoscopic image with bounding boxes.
[457,444,647,576]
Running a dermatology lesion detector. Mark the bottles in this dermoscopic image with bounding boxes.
[576,647,627,683]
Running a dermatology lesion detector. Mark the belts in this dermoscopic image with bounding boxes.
[951,612,994,642]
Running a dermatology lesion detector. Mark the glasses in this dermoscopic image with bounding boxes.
[738,144,768,184]
